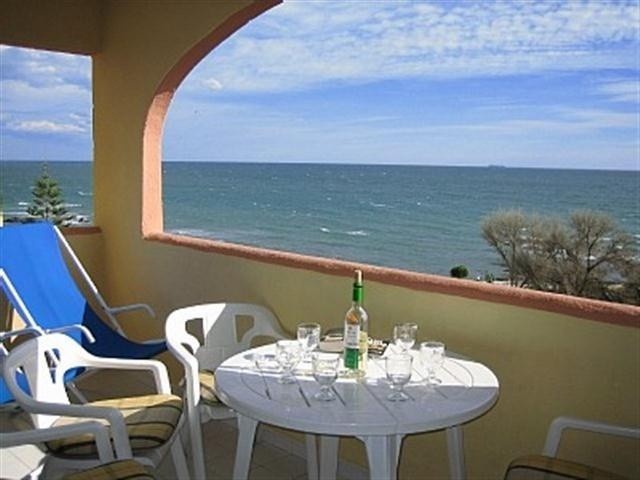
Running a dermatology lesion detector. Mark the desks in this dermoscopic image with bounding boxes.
[213,332,502,480]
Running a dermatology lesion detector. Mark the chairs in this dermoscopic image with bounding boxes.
[1,420,159,479]
[1,331,189,479]
[504,416,640,479]
[165,300,316,479]
[1,218,166,402]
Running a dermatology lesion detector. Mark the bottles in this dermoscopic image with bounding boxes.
[344,270,369,381]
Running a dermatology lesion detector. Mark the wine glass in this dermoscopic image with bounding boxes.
[385,320,446,402]
[275,322,341,400]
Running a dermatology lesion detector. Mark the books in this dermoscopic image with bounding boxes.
[320,327,362,352]
